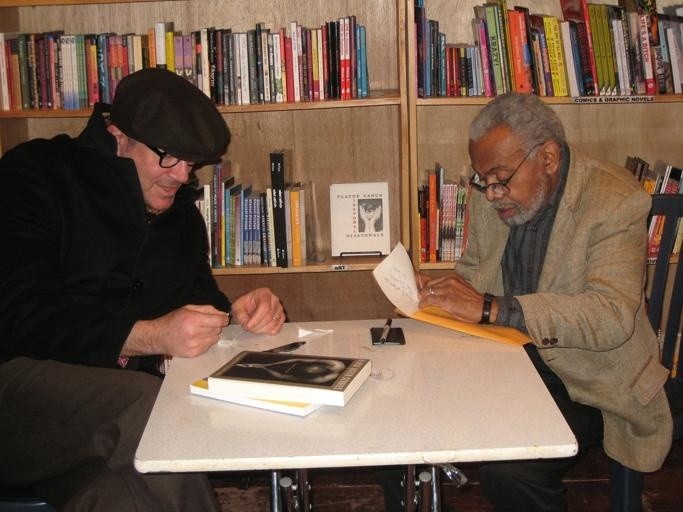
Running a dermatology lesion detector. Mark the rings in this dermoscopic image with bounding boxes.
[430,287,434,295]
[273,315,280,320]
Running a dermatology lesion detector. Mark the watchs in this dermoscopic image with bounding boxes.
[483,293,493,323]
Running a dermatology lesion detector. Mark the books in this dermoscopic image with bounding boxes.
[418,162,472,262]
[190,376,321,416]
[208,349,371,408]
[1,16,368,110]
[194,149,317,266]
[329,184,390,256]
[415,1,683,99]
[624,155,682,262]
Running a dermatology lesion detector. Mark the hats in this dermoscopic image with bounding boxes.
[106,66,233,168]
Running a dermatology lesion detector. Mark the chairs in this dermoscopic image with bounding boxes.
[0,474,70,512]
[442,193,683,510]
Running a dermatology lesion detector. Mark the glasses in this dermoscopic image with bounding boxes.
[468,141,547,199]
[137,140,209,170]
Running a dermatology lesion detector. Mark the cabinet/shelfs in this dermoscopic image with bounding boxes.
[406,0,683,380]
[0,1,409,324]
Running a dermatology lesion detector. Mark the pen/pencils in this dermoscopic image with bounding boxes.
[379,318,392,344]
[263,342,306,352]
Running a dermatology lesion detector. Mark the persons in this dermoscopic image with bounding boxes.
[378,93,674,511]
[2,67,286,512]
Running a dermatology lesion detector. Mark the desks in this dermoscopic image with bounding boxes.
[134,317,580,511]
